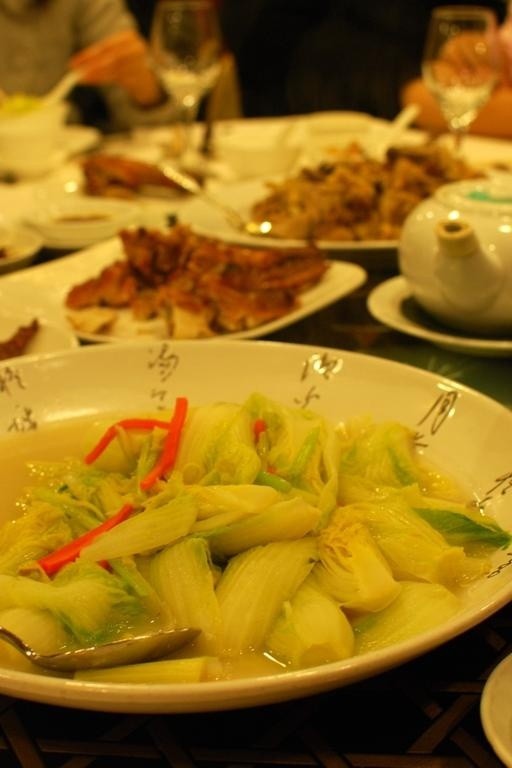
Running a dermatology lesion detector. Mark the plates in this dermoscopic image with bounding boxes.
[1,111,427,254]
[0,312,80,367]
[0,229,47,278]
[172,161,498,256]
[0,340,510,763]
[370,272,512,354]
[1,227,366,346]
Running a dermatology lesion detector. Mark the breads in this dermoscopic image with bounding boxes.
[80,150,200,201]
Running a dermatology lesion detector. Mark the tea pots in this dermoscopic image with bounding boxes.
[396,178,512,333]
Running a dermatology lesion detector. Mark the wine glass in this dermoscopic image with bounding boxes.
[420,8,505,153]
[150,1,232,164]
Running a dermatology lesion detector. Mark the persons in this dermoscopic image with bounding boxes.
[400,11,510,140]
[0,1,179,121]
